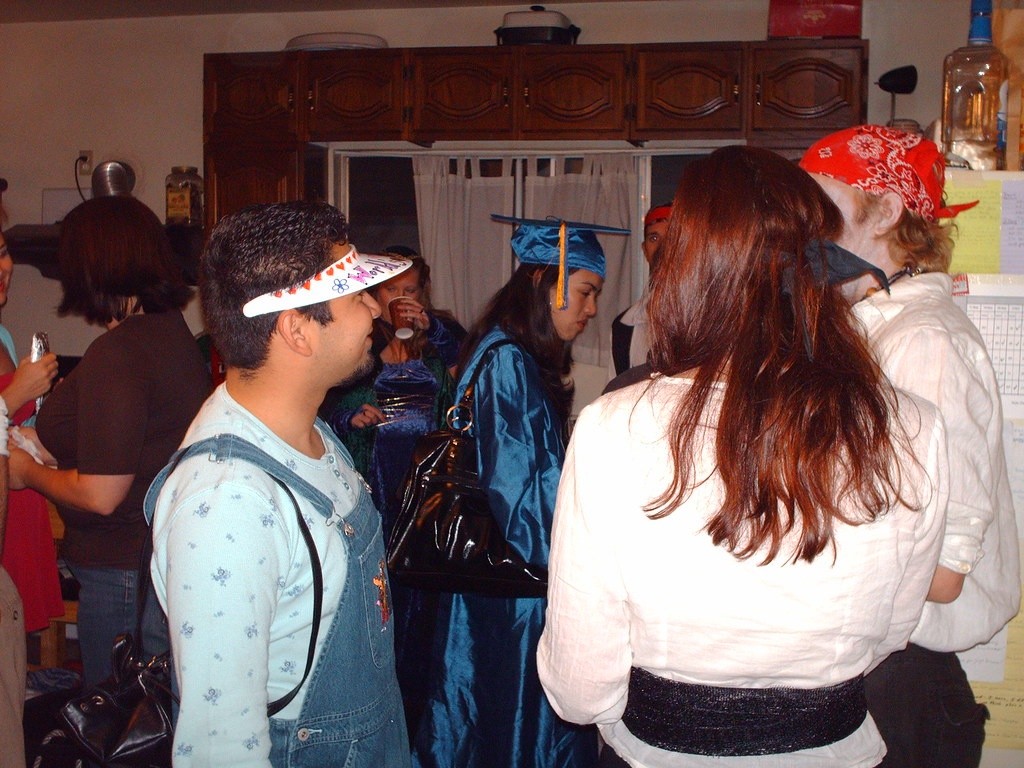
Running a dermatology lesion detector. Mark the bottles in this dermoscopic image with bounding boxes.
[940,0,1007,171]
[165,165,204,232]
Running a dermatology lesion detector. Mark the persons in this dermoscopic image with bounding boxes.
[534,147,949,768]
[8,195,214,685]
[144,200,413,768]
[611,200,673,375]
[394,215,628,768]
[802,124,1024,767]
[319,245,470,530]
[1,175,65,768]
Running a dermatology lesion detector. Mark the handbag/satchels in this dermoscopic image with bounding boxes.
[385,338,548,598]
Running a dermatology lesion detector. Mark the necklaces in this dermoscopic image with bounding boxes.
[864,263,927,296]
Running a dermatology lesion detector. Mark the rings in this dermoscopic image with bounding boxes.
[363,409,367,415]
[420,309,424,313]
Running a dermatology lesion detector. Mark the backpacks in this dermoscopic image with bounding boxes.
[34,444,322,768]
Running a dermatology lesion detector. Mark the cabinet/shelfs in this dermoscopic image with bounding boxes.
[203,142,329,249]
[630,36,869,148]
[407,43,640,151]
[203,47,407,142]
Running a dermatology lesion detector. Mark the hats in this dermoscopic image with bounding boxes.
[243,243,412,317]
[489,213,632,309]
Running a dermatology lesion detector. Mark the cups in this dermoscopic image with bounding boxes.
[388,296,415,340]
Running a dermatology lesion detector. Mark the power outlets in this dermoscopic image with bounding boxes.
[79,150,94,175]
